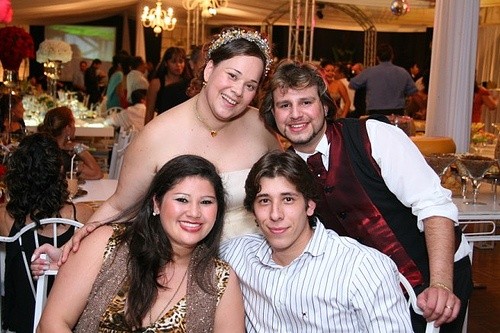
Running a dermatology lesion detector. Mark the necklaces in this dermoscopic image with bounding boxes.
[149,267,188,328]
[195,93,231,137]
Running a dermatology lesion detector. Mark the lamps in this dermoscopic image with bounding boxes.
[141,0,178,37]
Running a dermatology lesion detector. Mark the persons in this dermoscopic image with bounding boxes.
[143,46,189,126]
[37,106,104,180]
[263,58,473,333]
[0,27,473,333]
[347,43,418,115]
[472,69,496,124]
[30,30,285,280]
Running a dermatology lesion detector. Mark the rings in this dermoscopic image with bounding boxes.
[444,304,453,312]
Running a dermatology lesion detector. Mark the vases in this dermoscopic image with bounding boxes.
[44,61,61,81]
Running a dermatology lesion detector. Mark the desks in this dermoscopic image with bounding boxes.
[449,180,500,333]
[25,101,116,170]
[70,179,119,202]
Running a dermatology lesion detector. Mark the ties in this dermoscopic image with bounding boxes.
[307,151,327,185]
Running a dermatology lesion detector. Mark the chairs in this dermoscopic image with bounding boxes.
[0,217,84,333]
[94,104,135,181]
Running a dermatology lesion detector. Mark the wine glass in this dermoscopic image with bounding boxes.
[425,152,500,208]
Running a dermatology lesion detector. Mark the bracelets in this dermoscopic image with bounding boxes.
[73,144,88,155]
[430,282,452,292]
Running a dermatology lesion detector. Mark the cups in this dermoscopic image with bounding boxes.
[73,160,82,179]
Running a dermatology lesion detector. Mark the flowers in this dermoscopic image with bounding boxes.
[0,24,34,73]
[35,38,71,64]
